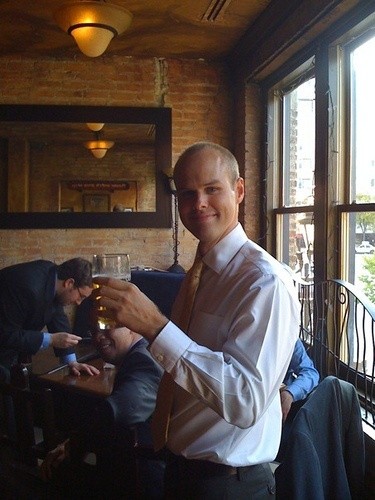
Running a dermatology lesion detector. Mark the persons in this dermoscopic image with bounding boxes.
[0,259,162,440]
[280,337,320,423]
[90,144,301,500]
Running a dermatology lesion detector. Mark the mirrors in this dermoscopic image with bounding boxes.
[0,104,174,230]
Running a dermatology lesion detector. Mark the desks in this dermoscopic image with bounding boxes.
[89,269,193,337]
[28,341,116,427]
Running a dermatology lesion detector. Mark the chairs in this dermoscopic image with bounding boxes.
[278,376,365,500]
[0,383,58,500]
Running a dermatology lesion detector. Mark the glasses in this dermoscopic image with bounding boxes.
[75,280,87,305]
[88,323,127,337]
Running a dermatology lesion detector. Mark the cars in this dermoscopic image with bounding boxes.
[355,243,375,254]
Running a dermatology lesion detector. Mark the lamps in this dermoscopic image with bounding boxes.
[161,167,189,274]
[54,1,133,58]
[86,133,113,158]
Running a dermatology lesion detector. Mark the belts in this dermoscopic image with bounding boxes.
[161,449,237,476]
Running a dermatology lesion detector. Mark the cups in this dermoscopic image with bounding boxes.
[92,253,131,334]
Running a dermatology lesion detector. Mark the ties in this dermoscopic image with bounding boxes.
[150,258,204,453]
